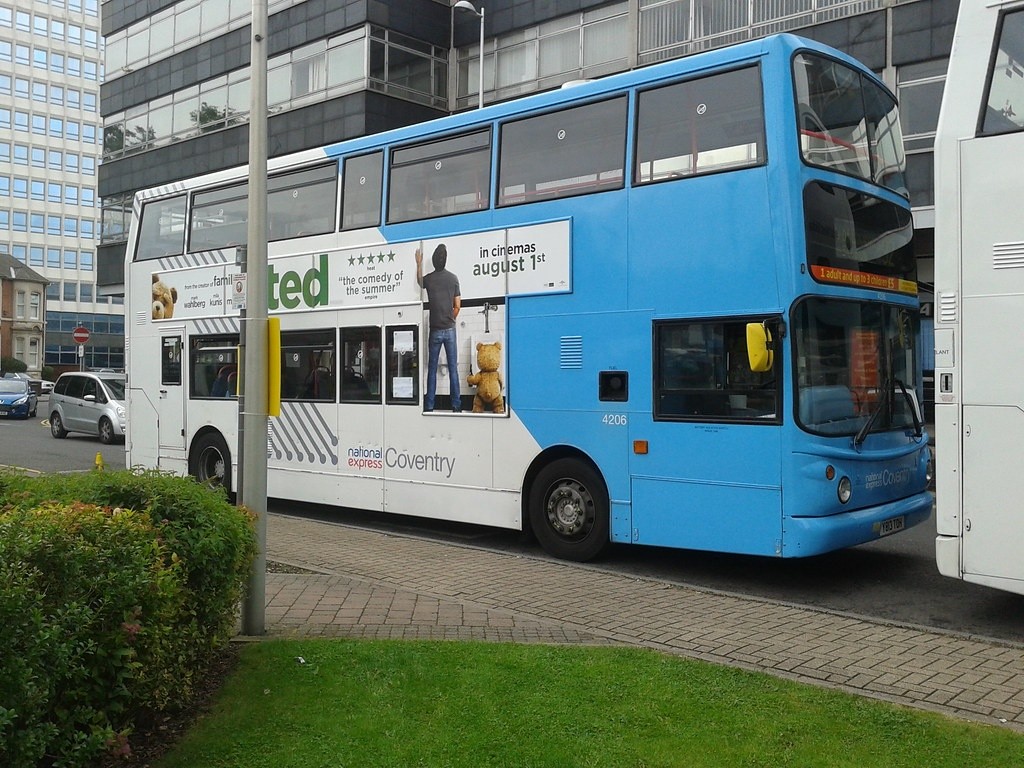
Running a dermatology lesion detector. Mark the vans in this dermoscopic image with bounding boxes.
[48,370,126,444]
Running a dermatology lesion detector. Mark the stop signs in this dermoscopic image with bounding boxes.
[73,328,91,344]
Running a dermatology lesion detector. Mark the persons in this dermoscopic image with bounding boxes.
[415,244,464,412]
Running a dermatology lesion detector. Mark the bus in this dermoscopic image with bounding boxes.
[120,30,937,570]
[930,0,1024,598]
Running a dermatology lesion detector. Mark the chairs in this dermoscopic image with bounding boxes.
[212,364,372,404]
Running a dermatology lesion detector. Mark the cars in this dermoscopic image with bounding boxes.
[0,379,38,419]
[4,372,55,394]
